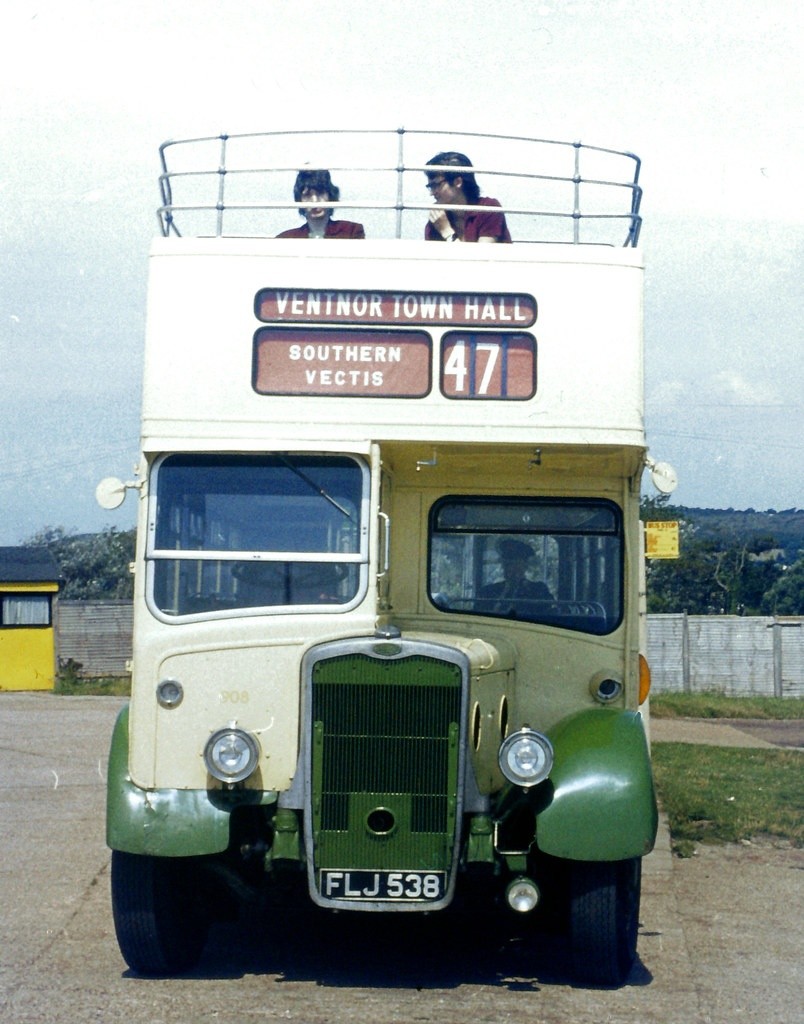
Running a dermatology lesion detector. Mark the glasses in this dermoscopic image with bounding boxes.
[427,178,450,192]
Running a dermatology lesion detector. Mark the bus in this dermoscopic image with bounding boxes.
[89,125,678,983]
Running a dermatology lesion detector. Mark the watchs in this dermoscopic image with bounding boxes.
[444,232,460,242]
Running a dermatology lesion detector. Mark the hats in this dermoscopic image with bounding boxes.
[496,540,535,563]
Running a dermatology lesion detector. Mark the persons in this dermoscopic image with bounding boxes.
[276,162,365,239]
[425,152,514,243]
[235,504,340,600]
[473,540,553,615]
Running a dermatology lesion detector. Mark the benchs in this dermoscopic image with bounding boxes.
[451,595,607,636]
